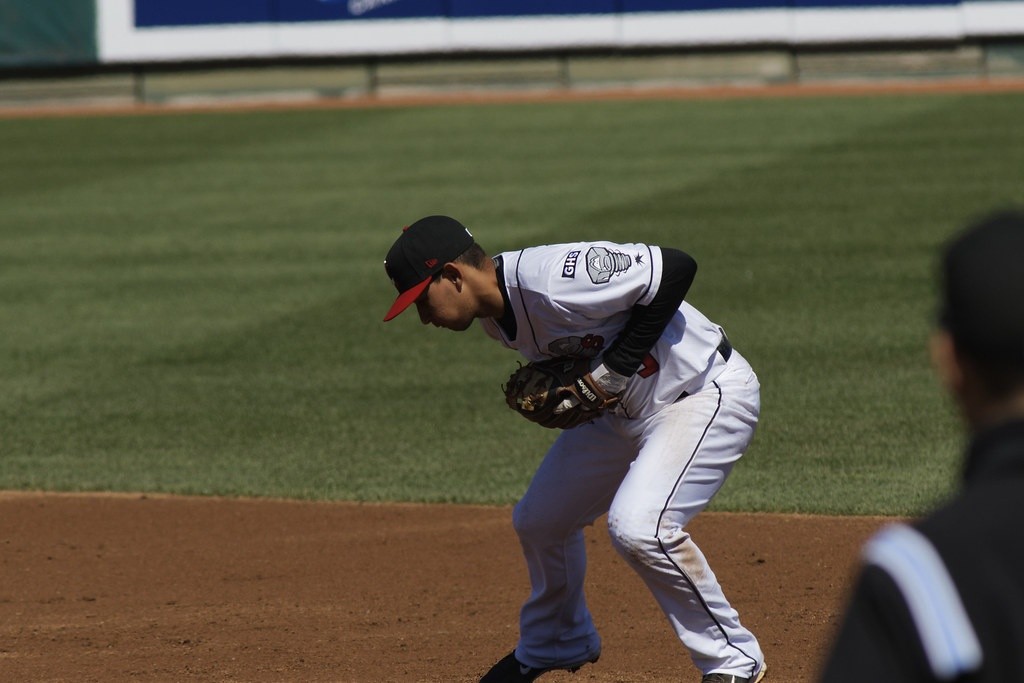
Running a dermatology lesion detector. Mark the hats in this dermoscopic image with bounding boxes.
[382,215,474,322]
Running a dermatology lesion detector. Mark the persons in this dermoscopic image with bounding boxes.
[381,216,769,683]
[809,199,1024,683]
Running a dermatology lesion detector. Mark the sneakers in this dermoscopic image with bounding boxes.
[478,646,601,683]
[701,662,767,683]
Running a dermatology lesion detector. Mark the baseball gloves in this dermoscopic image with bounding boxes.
[499,333,631,431]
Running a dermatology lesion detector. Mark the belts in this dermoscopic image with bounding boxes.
[674,327,732,403]
